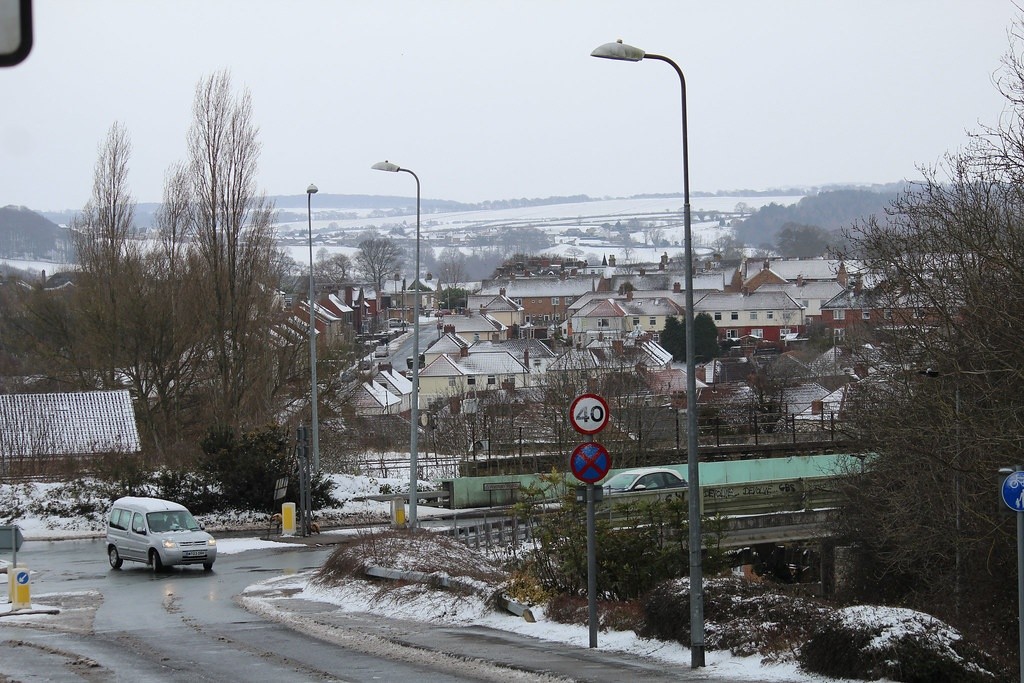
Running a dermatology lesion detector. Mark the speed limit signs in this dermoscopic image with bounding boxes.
[569,394,609,434]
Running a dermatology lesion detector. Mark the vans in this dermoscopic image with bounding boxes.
[106,496,217,575]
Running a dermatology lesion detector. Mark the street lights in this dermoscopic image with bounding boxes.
[372,160,418,529]
[307,184,320,472]
[591,39,706,670]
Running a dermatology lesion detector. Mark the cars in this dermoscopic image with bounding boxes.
[603,467,687,494]
[375,346,389,357]
[385,317,409,327]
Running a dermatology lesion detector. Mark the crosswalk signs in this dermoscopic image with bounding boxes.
[1002,471,1024,511]
